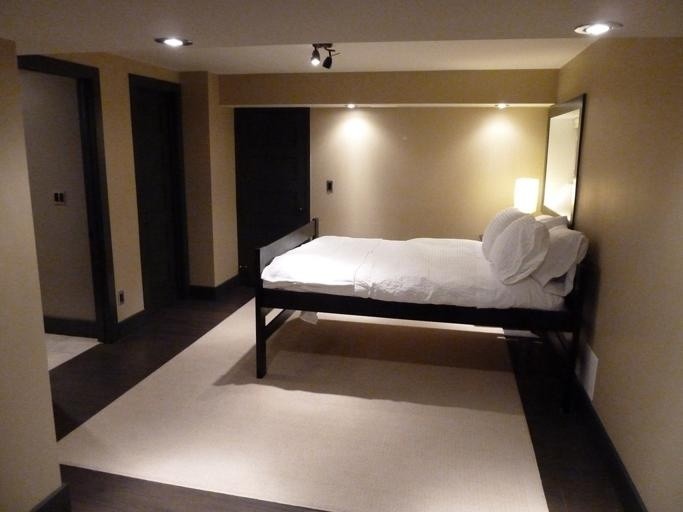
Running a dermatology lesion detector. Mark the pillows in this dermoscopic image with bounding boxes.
[481,208,590,295]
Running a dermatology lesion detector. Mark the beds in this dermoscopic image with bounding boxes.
[255,216,584,411]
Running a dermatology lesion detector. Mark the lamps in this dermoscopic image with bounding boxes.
[311,43,335,69]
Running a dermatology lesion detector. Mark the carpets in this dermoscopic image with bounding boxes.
[57,298,552,512]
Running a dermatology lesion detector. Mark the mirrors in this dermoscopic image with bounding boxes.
[539,92,586,226]
